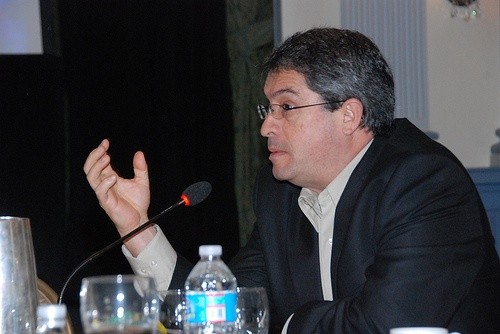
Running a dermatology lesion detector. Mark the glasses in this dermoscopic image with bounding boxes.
[257,100,345,119]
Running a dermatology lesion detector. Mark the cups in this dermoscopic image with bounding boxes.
[157,289,186,334]
[389,327,448,333]
[233,286,270,334]
[79,276,160,334]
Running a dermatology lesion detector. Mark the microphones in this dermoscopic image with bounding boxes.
[57,182,212,304]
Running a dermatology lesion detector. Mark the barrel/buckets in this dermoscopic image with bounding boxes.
[0,217,37,334]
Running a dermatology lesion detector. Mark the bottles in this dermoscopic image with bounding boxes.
[34,304,69,334]
[183,245,238,333]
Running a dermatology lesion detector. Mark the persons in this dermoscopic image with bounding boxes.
[84,27,500,334]
